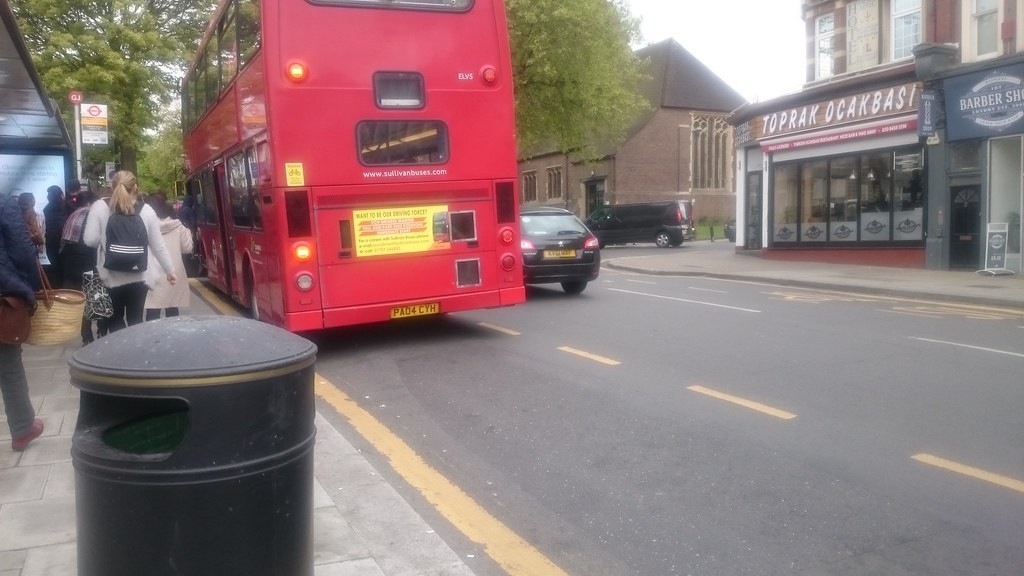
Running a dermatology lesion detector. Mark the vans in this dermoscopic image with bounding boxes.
[583,198,696,250]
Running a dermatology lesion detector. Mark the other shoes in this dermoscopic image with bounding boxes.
[12,417,44,452]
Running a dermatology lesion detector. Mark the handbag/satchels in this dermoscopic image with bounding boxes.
[81,269,116,320]
[1,297,33,345]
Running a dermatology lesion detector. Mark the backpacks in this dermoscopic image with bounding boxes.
[103,197,152,274]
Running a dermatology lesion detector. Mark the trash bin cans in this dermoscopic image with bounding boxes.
[69,314,320,576]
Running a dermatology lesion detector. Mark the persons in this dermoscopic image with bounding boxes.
[229,186,261,226]
[80,171,198,346]
[0,182,99,451]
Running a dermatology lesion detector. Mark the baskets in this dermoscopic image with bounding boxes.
[27,255,86,347]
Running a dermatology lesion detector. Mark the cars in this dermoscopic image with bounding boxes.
[518,204,601,292]
[723,220,736,243]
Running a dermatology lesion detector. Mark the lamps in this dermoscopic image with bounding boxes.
[848,169,857,179]
[867,168,876,178]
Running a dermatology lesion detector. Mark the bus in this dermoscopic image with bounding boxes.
[175,0,528,334]
